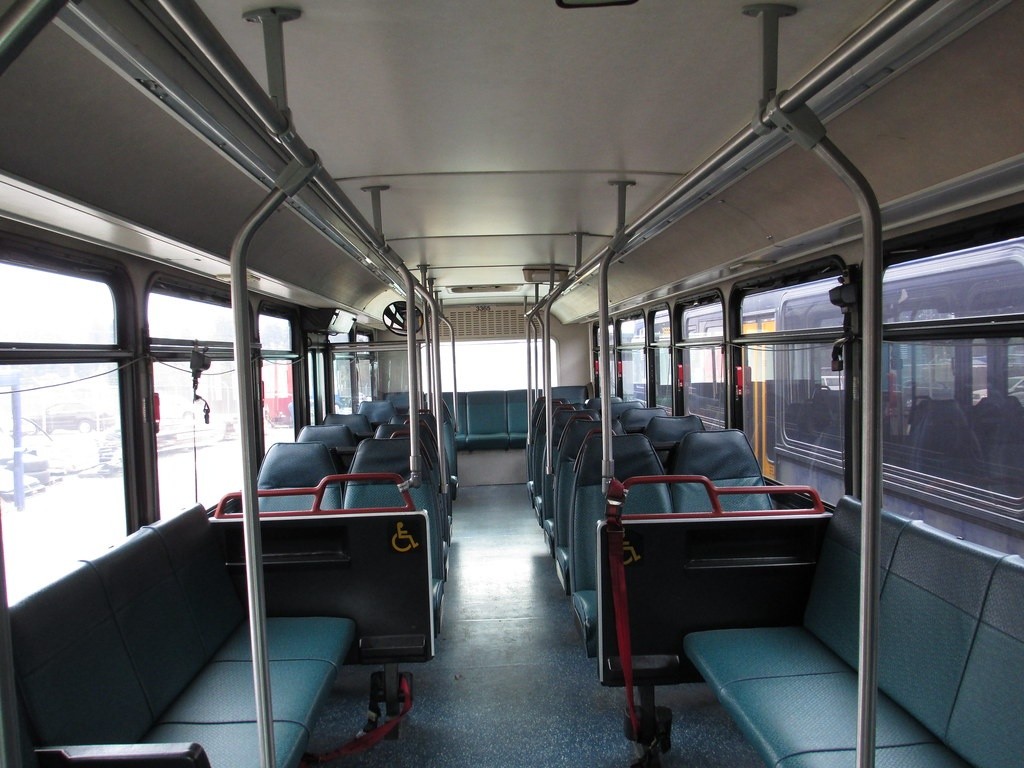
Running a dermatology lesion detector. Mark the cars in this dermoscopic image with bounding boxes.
[820,375,844,391]
[971,375,1024,408]
[8,398,116,438]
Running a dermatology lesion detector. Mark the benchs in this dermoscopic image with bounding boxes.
[690,497,1024,768]
[385,385,589,453]
[9,505,354,768]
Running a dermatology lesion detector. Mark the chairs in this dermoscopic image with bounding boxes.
[250,396,776,657]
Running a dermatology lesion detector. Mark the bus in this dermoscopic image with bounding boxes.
[260,359,296,425]
[628,237,1024,565]
